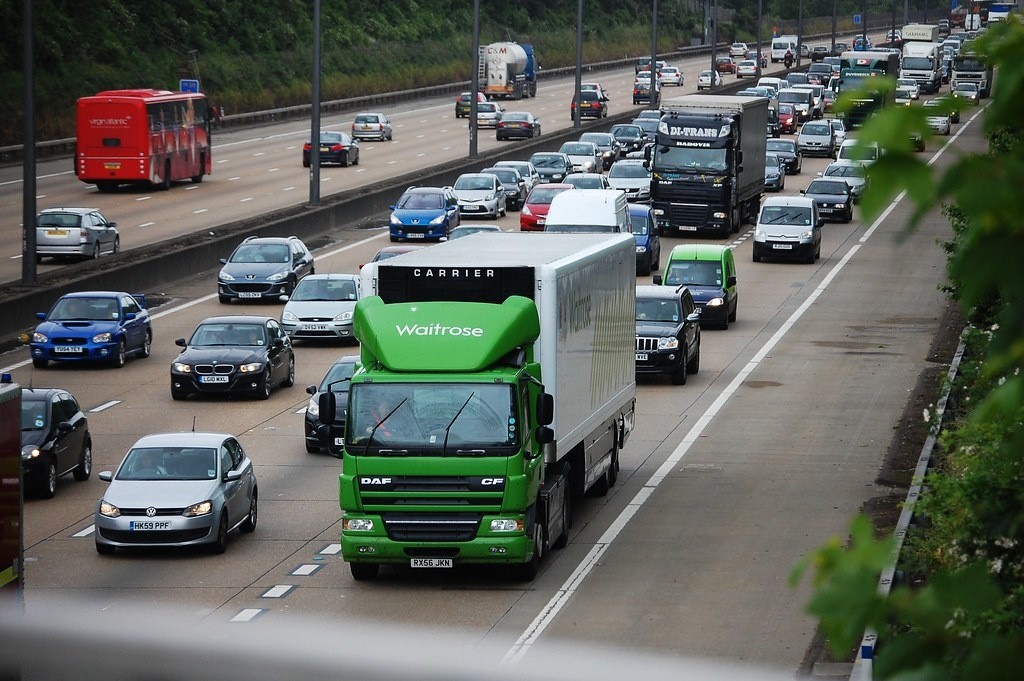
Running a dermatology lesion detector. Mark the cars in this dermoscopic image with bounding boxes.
[451,2,1018,276]
[93,417,259,557]
[359,245,427,269]
[304,354,361,460]
[36,207,120,263]
[388,184,460,244]
[303,130,360,168]
[29,289,154,371]
[20,365,93,500]
[217,235,316,305]
[169,315,296,402]
[352,111,393,142]
[438,224,503,242]
[278,272,361,347]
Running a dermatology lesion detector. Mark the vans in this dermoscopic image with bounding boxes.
[652,243,738,331]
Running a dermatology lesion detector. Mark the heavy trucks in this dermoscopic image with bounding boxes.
[477,41,539,101]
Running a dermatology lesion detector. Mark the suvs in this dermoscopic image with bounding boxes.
[633,285,703,386]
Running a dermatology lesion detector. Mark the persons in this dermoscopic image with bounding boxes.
[667,266,689,284]
[210,104,225,131]
[706,153,727,170]
[103,303,119,319]
[139,453,167,476]
[365,401,406,440]
[238,248,266,263]
[341,283,352,299]
[784,50,794,66]
[206,331,222,344]
[60,302,87,318]
[245,330,259,345]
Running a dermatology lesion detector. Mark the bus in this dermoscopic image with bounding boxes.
[73,88,225,194]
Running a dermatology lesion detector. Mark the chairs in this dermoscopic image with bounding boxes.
[107,303,117,321]
[55,217,63,225]
[208,331,224,343]
[140,457,161,476]
[309,285,325,299]
[247,332,262,345]
[658,303,674,321]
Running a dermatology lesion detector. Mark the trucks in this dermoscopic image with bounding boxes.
[338,231,639,586]
[642,94,770,238]
[835,50,899,132]
[902,40,943,94]
